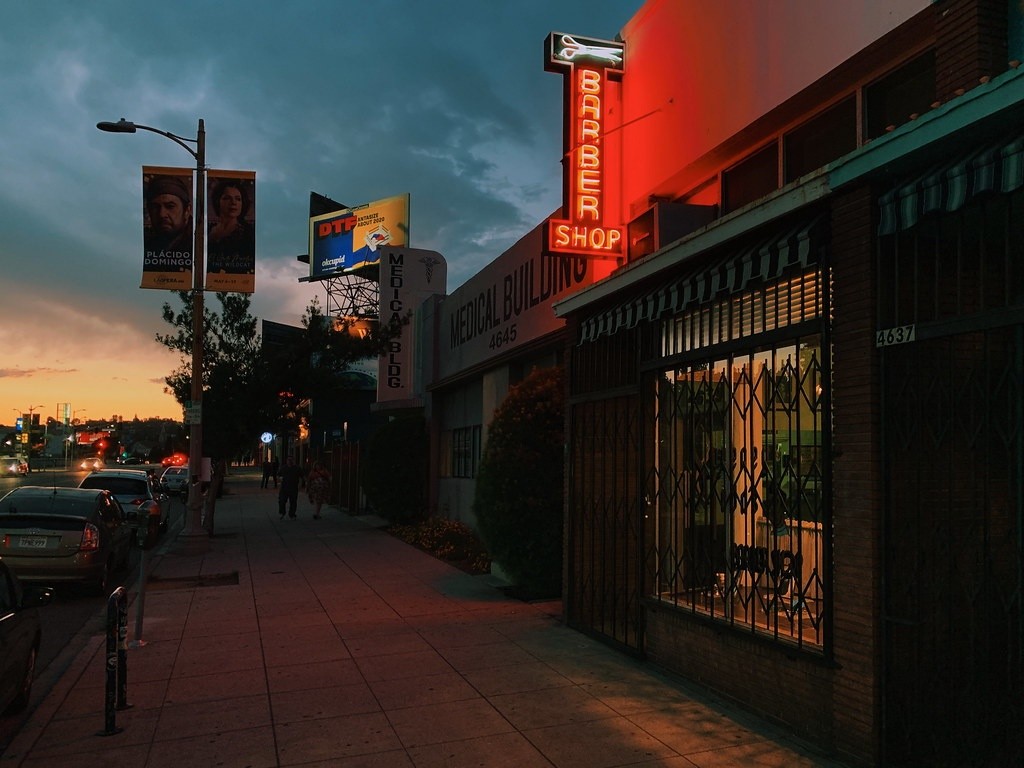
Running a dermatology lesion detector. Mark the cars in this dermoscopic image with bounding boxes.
[0,557,55,717]
[0,468,140,596]
[0,456,29,477]
[117,455,142,465]
[161,455,184,468]
[77,468,179,546]
[79,456,104,472]
[160,465,189,496]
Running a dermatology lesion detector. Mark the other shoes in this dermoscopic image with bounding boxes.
[279,512,322,520]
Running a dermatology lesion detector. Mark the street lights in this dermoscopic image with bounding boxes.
[96,114,217,546]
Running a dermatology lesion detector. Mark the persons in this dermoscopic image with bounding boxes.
[306,461,333,520]
[276,456,306,521]
[261,456,280,490]
